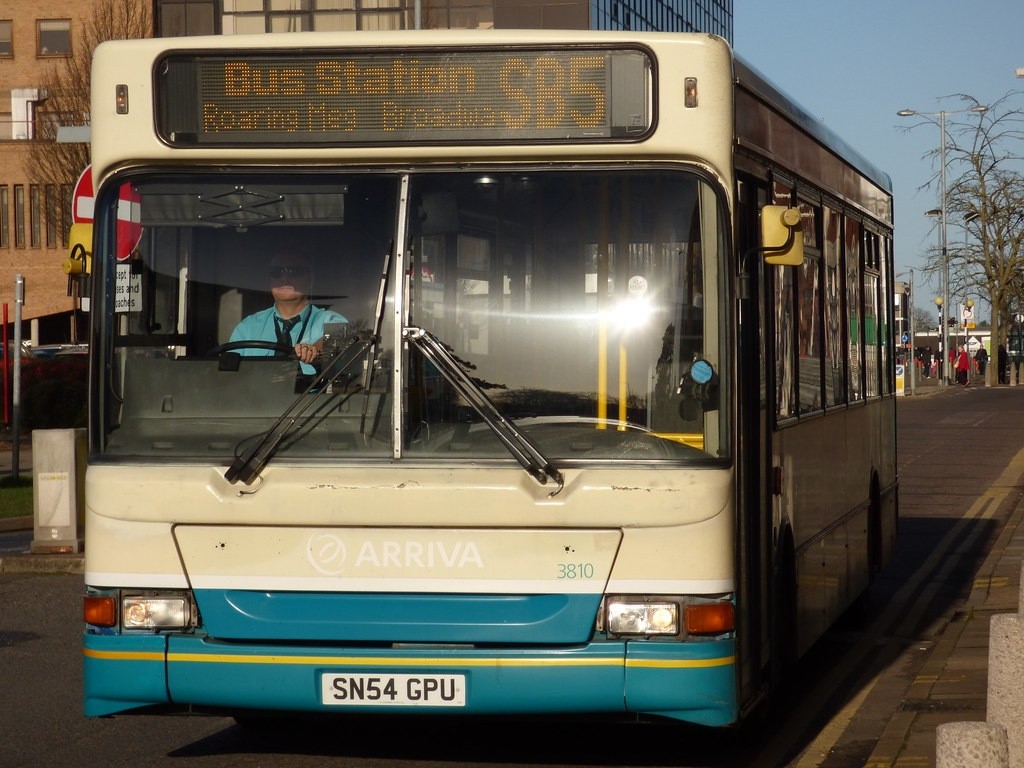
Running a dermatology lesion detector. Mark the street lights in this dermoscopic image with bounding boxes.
[962,210,981,383]
[934,297,944,386]
[898,106,989,386]
[965,300,974,385]
[924,209,943,384]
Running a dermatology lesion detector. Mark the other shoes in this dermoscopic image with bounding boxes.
[922,374,931,378]
[999,383,1001,384]
[961,381,971,388]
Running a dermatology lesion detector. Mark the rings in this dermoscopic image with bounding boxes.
[302,346,308,351]
[307,349,313,352]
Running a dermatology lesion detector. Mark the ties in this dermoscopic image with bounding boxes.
[275,315,301,356]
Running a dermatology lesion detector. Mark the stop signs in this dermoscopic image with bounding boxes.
[71,164,144,261]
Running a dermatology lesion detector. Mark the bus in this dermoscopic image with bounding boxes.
[66,21,894,728]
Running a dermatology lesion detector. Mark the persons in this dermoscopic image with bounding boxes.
[226,243,351,395]
[896,344,988,387]
[998,344,1009,384]
